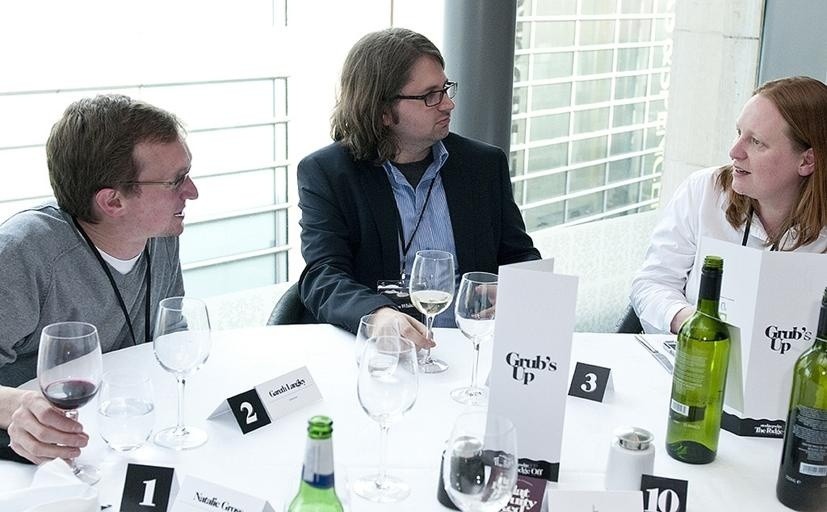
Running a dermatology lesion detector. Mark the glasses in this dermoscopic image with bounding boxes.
[389,80,458,106]
[95,167,189,199]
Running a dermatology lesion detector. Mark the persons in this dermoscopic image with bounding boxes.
[628,76,827,334]
[0,93,199,463]
[296,27,540,357]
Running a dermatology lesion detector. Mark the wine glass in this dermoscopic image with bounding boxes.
[444,411,517,511]
[37,321,102,485]
[355,313,418,504]
[409,249,456,375]
[154,295,213,449]
[452,269,499,406]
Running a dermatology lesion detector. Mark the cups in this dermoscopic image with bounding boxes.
[97,369,157,453]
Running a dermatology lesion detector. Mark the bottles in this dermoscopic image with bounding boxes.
[777,285,827,512]
[667,255,732,464]
[287,416,344,511]
